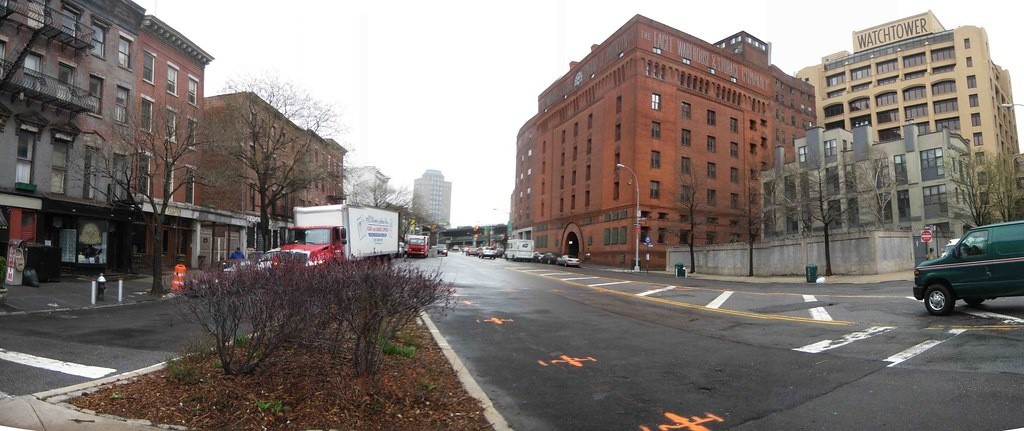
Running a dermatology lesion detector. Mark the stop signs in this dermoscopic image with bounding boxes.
[920,230,933,243]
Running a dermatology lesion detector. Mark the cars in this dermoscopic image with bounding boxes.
[192,259,255,294]
[429,242,507,260]
[256,249,282,270]
[531,252,546,263]
[557,255,581,267]
[398,242,405,258]
[540,253,563,263]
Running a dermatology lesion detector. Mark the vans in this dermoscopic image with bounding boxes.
[504,239,534,261]
[913,221,1024,316]
[942,238,984,258]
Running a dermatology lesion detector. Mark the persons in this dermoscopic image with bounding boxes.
[631,256,642,271]
[230,247,244,260]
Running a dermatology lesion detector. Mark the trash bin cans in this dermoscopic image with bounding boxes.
[805,263,818,283]
[675,262,685,276]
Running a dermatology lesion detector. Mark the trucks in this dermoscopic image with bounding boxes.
[269,203,399,277]
[406,238,428,258]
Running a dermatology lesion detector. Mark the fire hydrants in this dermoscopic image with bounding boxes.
[97,273,108,302]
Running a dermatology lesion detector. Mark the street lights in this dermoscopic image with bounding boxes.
[616,163,642,271]
